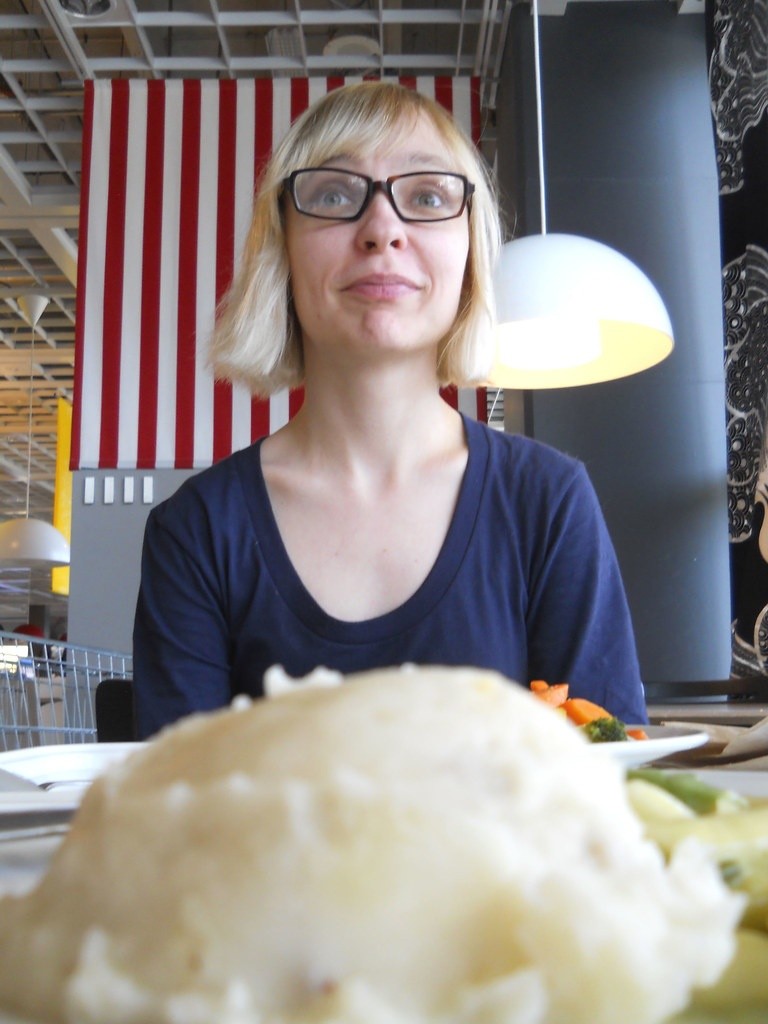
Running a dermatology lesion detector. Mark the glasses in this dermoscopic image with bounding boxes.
[282,167,475,222]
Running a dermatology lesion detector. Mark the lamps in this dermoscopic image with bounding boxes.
[440,0,675,390]
[1,294,73,567]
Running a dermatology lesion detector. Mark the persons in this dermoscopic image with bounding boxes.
[130,86,646,726]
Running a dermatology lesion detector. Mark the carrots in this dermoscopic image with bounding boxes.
[526,678,648,741]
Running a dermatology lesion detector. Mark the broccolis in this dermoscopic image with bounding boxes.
[578,716,628,743]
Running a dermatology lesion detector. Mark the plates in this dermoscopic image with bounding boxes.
[0,741,150,792]
[591,724,709,770]
[660,768,768,799]
